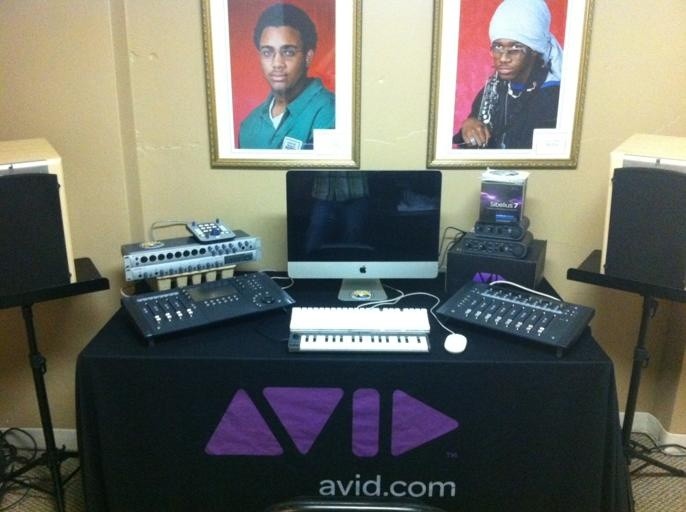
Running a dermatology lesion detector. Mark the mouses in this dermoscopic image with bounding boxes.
[443,333,469,354]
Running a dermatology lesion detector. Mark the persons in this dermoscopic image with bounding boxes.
[452,0,563,148]
[238,3,335,150]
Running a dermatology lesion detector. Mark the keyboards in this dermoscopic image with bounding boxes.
[288,306,432,333]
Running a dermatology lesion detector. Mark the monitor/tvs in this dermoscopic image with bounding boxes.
[286,170,442,302]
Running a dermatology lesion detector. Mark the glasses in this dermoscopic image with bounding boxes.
[489,44,527,59]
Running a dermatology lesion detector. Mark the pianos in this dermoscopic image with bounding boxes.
[289,333,431,353]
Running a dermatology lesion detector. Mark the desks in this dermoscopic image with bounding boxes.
[74,271,635,511]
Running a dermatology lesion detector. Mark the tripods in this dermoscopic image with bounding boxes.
[0,306,83,512]
[621,294,686,477]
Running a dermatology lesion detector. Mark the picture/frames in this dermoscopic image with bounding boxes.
[199,0,363,170]
[425,0,595,169]
